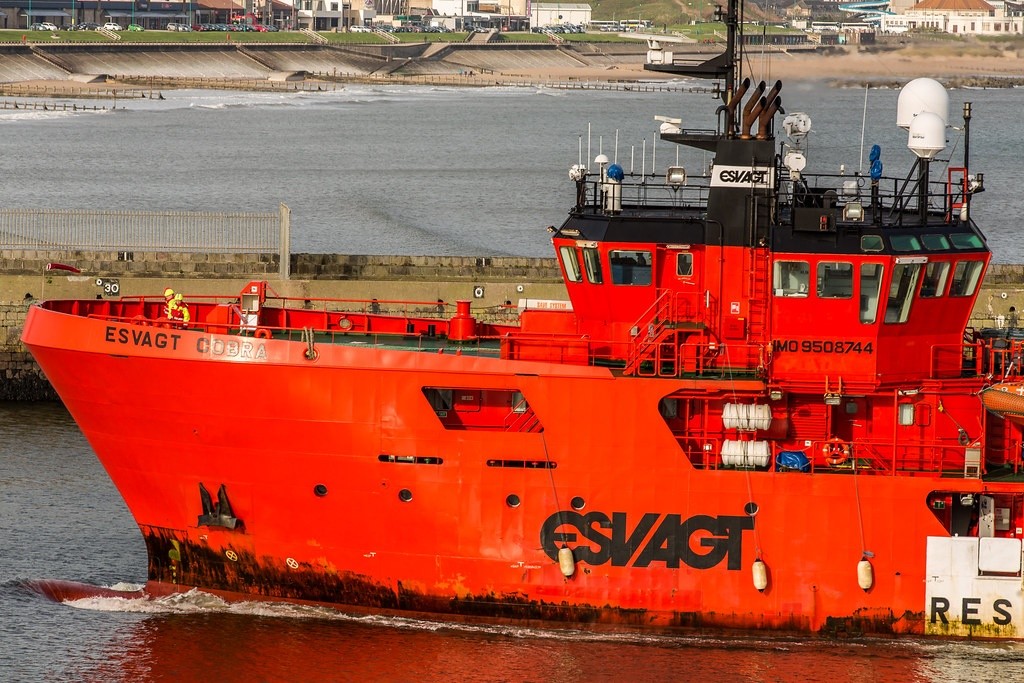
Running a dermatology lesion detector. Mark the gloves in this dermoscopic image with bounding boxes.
[183,324,188,328]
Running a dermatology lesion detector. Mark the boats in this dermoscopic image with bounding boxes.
[20,0,1024,662]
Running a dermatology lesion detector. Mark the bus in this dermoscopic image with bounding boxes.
[590,20,653,32]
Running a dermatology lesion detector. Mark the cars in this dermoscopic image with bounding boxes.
[103,22,123,32]
[167,22,280,32]
[350,23,489,35]
[30,21,103,32]
[128,23,145,33]
[531,22,586,35]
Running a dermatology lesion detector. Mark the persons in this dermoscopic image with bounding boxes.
[164,288,190,330]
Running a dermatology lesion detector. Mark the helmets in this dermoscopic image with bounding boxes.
[164,288,174,296]
[174,293,183,300]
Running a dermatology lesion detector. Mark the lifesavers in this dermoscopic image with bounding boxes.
[823,437,848,464]
